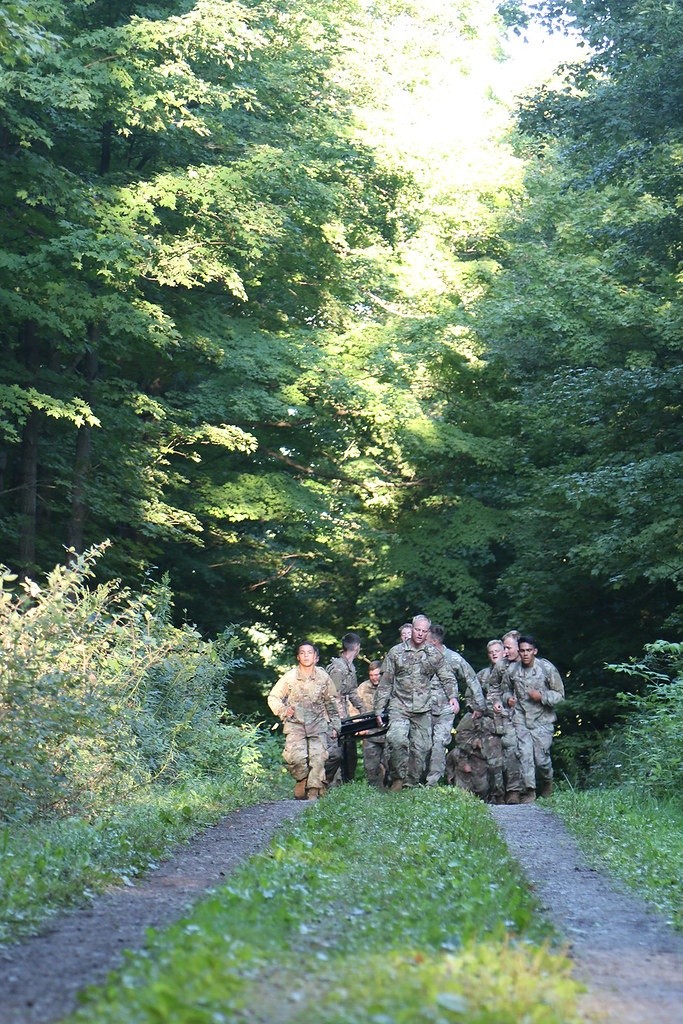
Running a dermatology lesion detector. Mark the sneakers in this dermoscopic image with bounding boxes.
[308,787,320,802]
[540,781,552,796]
[293,777,309,798]
[496,796,505,805]
[390,779,403,791]
[506,791,520,803]
[520,789,536,804]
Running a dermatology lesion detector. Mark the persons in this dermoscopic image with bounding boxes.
[267,642,344,802]
[501,635,564,804]
[358,660,382,787]
[398,623,412,642]
[465,638,504,804]
[422,625,487,785]
[313,646,343,796]
[486,630,523,806]
[374,614,460,793]
[445,712,491,804]
[326,632,362,784]
